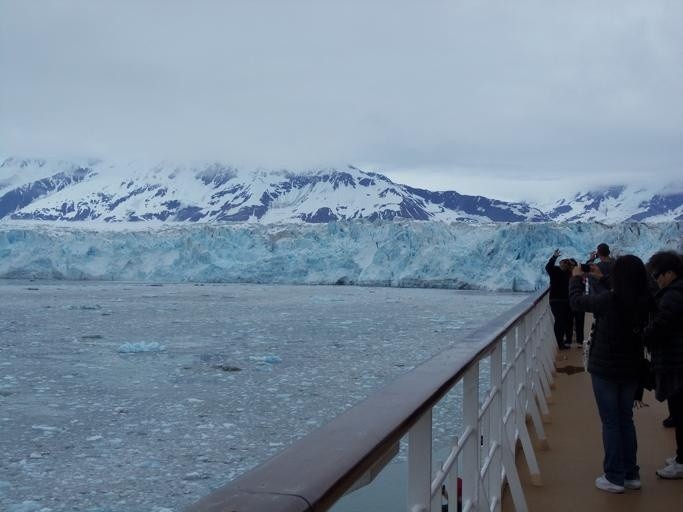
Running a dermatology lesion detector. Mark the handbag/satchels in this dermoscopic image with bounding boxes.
[643,349,655,392]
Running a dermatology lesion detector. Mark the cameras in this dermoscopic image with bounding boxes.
[581,264,590,272]
[596,254,600,258]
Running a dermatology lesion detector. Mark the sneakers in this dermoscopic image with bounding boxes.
[662,414,674,429]
[564,343,584,350]
[592,471,642,495]
[655,456,682,479]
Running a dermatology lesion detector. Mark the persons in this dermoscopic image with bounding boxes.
[544,242,683,494]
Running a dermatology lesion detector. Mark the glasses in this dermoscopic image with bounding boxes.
[651,272,661,281]
